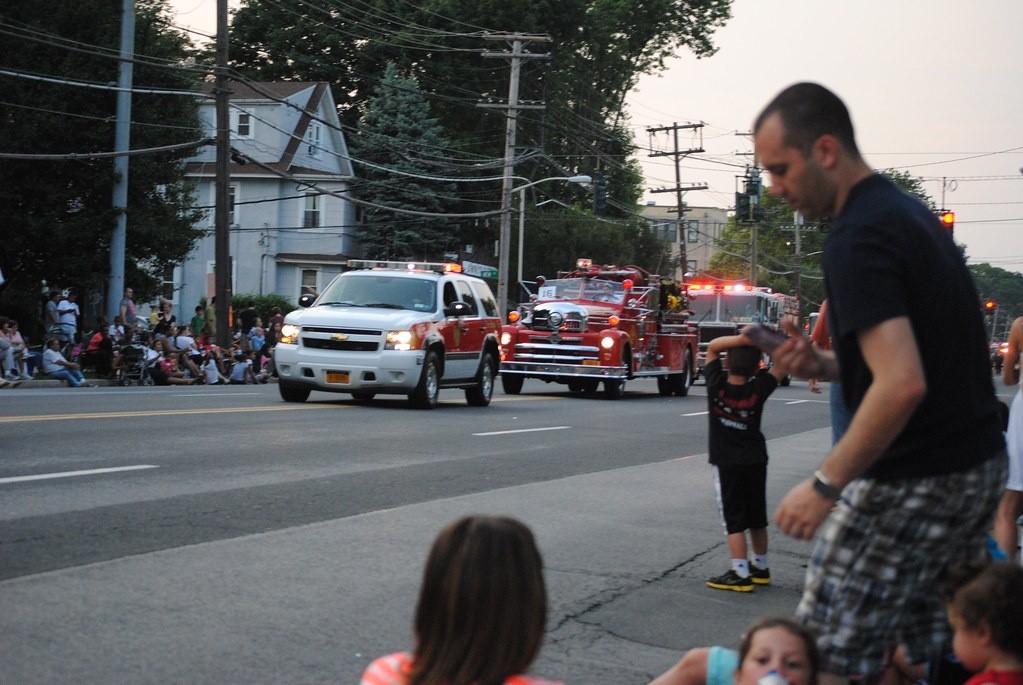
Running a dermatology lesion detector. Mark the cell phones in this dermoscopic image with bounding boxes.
[743,324,791,353]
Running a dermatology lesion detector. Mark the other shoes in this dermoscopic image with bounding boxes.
[80,381,94,387]
[809,384,822,394]
[21,353,35,361]
[6,374,21,380]
[21,373,34,380]
[12,346,24,355]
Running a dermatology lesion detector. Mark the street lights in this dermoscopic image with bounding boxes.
[497,176,592,326]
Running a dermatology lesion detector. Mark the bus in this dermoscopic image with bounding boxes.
[804,313,820,335]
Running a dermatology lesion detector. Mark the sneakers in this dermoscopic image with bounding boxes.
[707,569,755,591]
[748,560,772,584]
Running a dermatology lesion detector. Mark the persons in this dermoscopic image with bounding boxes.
[646,78,1023,685]
[700,323,789,591]
[358,515,568,685]
[119,288,284,385]
[0,287,125,387]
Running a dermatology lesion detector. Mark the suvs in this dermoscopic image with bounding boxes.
[275,269,503,408]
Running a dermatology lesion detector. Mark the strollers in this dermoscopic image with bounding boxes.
[120,340,152,386]
[42,327,72,361]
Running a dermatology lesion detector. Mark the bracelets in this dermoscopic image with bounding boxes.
[811,469,840,503]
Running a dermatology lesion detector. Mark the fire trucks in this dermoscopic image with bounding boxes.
[500,258,699,400]
[683,282,799,388]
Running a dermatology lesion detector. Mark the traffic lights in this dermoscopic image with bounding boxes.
[985,299,992,315]
[943,212,954,236]
